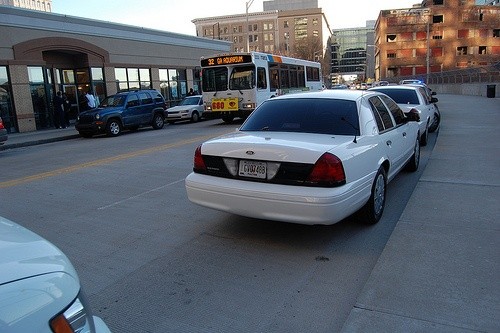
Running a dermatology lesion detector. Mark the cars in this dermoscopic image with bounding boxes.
[0,116,10,143]
[186,89,422,226]
[0,216,111,333]
[335,80,426,90]
[366,83,442,146]
[167,95,206,123]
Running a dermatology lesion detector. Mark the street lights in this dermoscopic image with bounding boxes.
[355,44,382,83]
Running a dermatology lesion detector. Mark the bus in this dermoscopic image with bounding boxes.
[201,52,324,123]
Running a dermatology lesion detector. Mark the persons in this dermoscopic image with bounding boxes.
[52,92,70,129]
[187,88,195,96]
[79,90,95,112]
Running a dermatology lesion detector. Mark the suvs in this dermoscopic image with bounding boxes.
[76,88,169,137]
